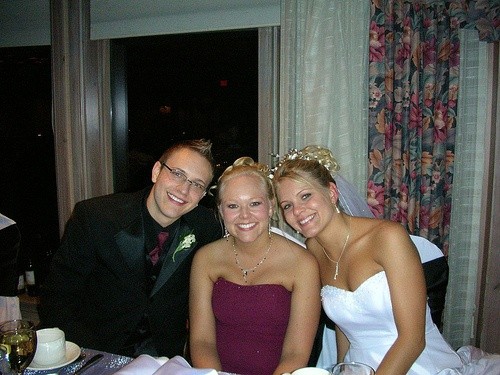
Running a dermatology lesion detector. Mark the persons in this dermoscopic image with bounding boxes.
[38,138,222,370]
[189,157,322,375]
[273,146,464,375]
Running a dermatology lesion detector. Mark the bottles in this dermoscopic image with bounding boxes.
[25,258,36,298]
[0,345,11,375]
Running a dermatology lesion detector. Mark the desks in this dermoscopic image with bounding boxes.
[0,344,134,375]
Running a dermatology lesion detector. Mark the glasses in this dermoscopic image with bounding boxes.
[163,162,206,195]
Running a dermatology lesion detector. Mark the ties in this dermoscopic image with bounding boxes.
[144,231,170,267]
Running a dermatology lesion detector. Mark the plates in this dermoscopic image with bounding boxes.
[26,341,82,370]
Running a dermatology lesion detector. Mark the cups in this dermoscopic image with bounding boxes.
[33,327,66,365]
[290,367,331,375]
[332,361,376,375]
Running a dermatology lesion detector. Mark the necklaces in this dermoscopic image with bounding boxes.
[232,233,273,283]
[322,215,351,280]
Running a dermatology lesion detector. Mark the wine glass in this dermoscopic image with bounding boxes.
[0,319,37,375]
